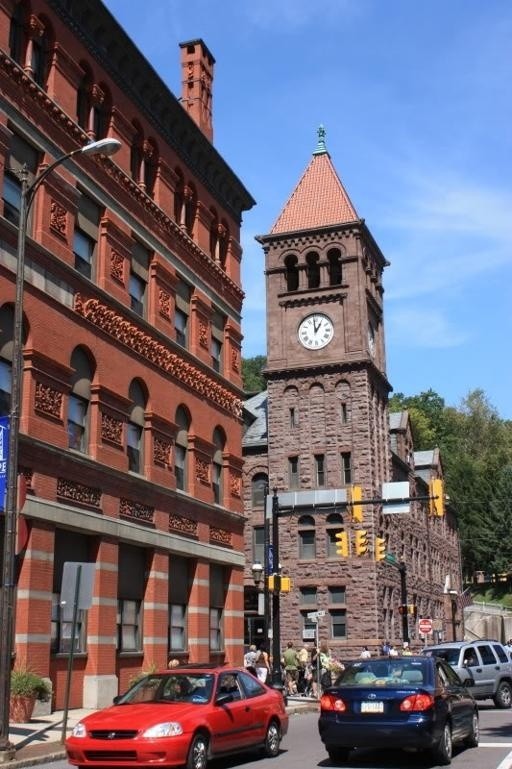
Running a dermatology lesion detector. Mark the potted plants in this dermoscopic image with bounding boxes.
[129,662,163,703]
[7,670,54,725]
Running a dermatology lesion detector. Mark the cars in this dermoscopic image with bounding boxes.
[315,654,478,764]
[65,660,290,769]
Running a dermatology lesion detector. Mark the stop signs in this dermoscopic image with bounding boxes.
[419,619,433,634]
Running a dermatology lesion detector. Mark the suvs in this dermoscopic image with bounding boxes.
[417,638,512,709]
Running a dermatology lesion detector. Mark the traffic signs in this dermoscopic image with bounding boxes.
[306,610,326,619]
[384,553,396,563]
[310,617,323,624]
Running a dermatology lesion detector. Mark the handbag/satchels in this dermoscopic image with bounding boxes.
[321,671,331,687]
[267,674,272,684]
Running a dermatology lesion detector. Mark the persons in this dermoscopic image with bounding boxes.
[168,657,181,696]
[402,647,411,656]
[359,645,371,658]
[243,641,331,698]
[389,646,398,657]
[383,642,392,655]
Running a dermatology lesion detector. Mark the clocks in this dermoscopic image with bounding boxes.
[296,312,335,351]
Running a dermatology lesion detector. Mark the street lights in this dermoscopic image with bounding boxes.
[250,563,286,705]
[1,138,124,764]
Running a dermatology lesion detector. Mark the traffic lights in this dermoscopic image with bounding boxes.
[335,531,349,558]
[357,529,368,555]
[375,537,387,562]
[398,605,408,615]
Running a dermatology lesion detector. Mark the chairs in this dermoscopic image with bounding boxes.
[353,670,424,685]
